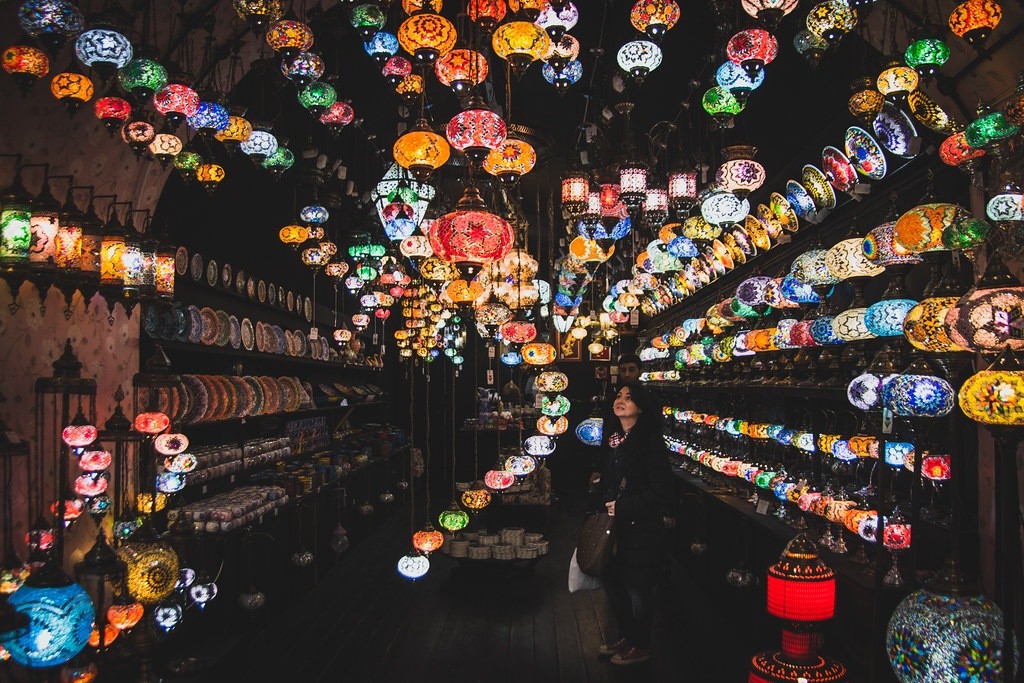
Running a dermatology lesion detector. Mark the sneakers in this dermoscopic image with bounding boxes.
[599,634,630,655]
[610,644,651,665]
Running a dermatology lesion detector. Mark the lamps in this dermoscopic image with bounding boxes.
[0,0,1024,683]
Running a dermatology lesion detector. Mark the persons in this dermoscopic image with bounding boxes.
[594,379,678,664]
[590,354,644,486]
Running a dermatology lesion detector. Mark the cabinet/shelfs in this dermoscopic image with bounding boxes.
[141,249,411,549]
[634,162,981,599]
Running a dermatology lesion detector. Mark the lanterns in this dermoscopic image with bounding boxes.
[0,0,1024,683]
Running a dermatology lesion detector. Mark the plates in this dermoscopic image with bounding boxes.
[134,243,383,418]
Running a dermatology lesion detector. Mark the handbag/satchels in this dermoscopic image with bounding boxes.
[567,549,605,592]
[577,512,619,576]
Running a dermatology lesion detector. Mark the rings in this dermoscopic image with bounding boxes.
[610,510,612,514]
